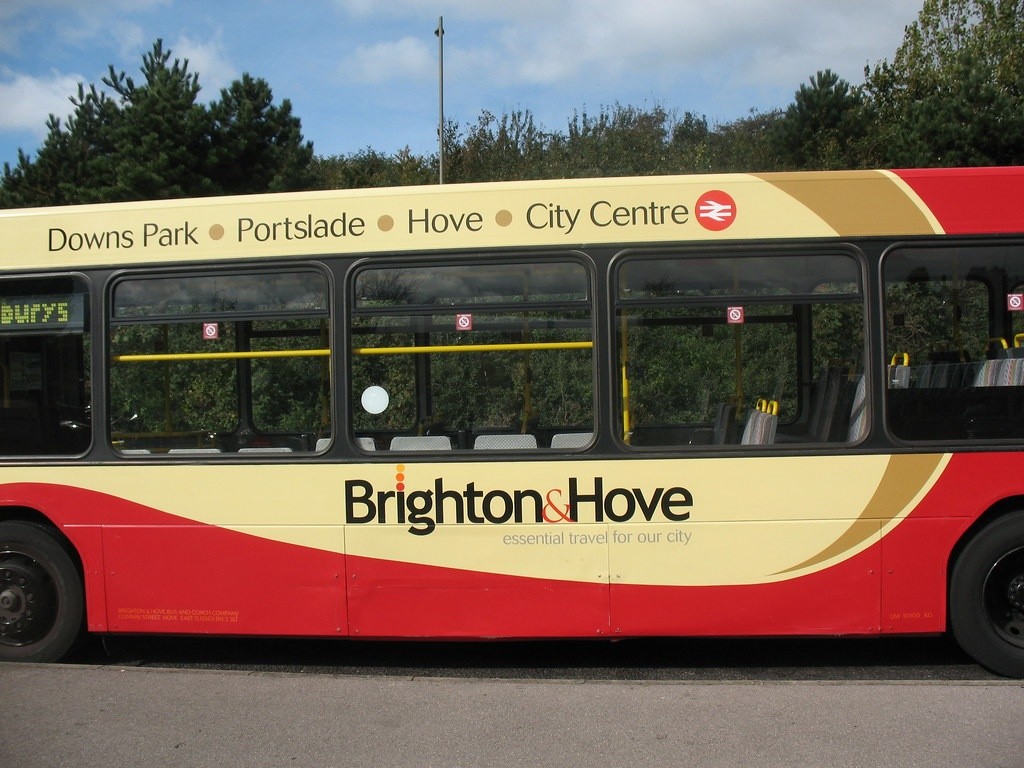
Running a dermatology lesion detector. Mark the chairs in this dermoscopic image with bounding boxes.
[121,432,593,456]
[688,333,1024,445]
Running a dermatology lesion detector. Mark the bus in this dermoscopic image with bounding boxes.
[0,164,1024,678]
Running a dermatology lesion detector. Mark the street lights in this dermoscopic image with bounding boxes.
[435,16,445,184]
